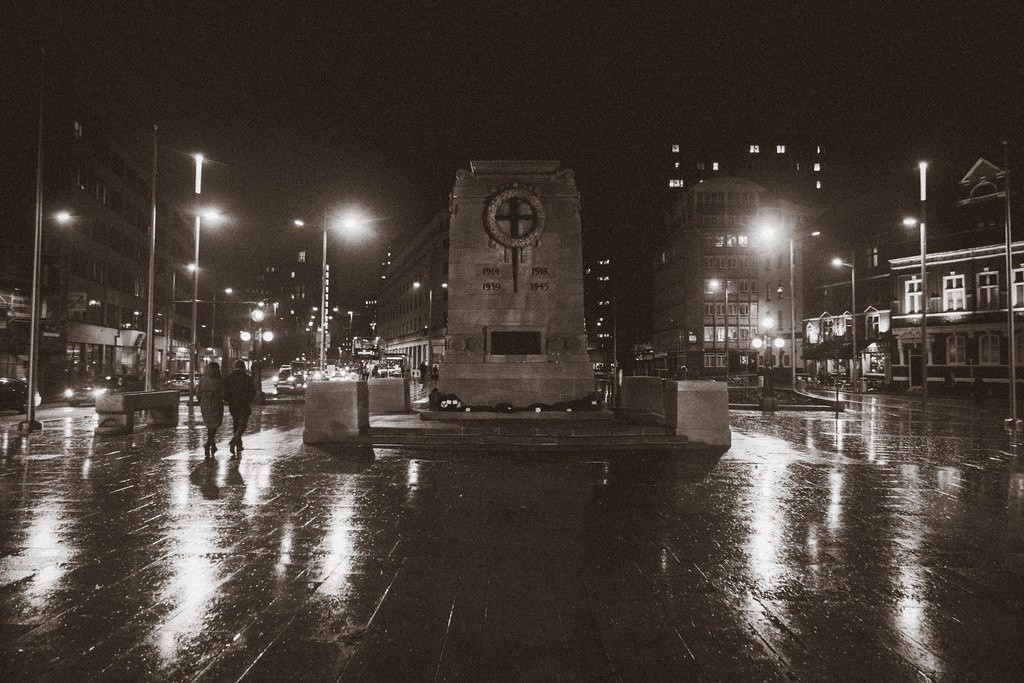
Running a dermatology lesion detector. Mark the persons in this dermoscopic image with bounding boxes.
[222,360,256,455]
[419,360,438,380]
[194,359,226,456]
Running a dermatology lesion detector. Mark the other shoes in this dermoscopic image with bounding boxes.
[203,444,210,455]
[228,441,236,455]
[211,446,217,455]
[237,442,244,451]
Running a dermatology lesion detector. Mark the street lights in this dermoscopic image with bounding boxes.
[765,226,822,389]
[833,259,859,391]
[294,217,365,371]
[902,159,930,419]
[751,312,786,396]
[709,280,732,381]
[239,308,274,391]
[184,147,228,412]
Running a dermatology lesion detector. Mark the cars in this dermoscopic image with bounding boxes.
[272,362,361,395]
[0,376,41,414]
[166,373,204,394]
[67,374,150,407]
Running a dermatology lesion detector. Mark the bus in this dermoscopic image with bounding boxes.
[350,336,389,378]
[384,353,411,378]
